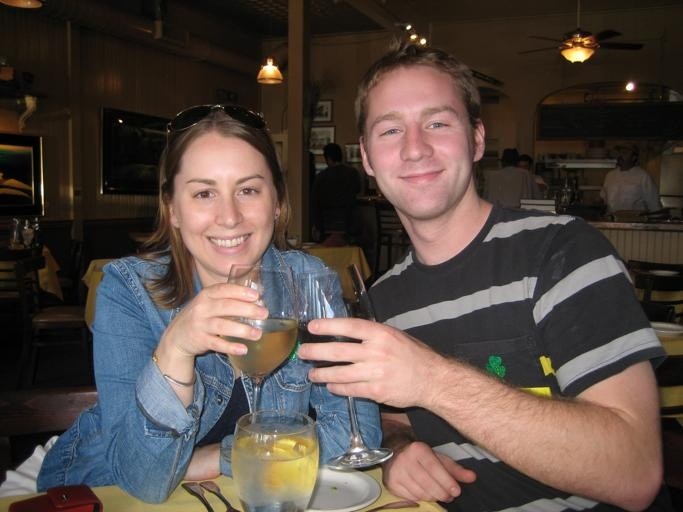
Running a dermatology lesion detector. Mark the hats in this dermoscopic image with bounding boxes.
[616,141,641,155]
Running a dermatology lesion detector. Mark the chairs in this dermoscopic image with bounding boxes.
[628,260,683,324]
[1,227,112,391]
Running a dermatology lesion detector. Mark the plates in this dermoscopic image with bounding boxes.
[303,465,382,512]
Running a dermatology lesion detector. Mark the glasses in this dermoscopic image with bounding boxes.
[166,104,265,135]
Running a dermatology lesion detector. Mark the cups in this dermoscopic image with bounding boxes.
[231,407,320,511]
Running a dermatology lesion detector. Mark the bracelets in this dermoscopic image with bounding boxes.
[149,349,197,387]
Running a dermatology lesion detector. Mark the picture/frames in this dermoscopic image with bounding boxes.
[309,98,363,164]
[0,133,48,216]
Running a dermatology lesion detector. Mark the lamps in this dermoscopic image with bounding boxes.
[254,10,283,85]
[560,47,596,64]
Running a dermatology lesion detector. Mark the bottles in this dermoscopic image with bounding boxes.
[11,217,41,249]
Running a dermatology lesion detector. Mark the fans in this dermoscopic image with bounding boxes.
[516,0,646,56]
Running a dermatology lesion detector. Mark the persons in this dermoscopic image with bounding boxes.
[583,140,664,221]
[480,147,544,214]
[306,142,365,245]
[0,100,384,503]
[516,153,550,195]
[293,39,667,511]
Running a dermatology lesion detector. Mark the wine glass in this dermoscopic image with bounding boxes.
[292,262,394,471]
[223,262,299,462]
